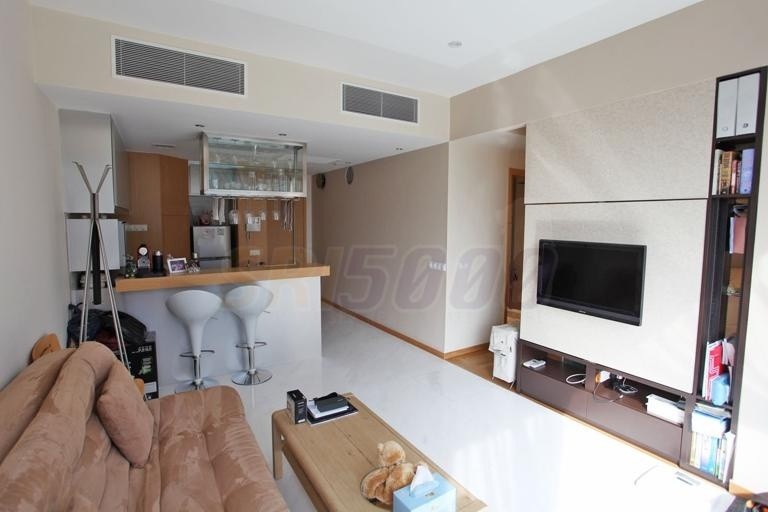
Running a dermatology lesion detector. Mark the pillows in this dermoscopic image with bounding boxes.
[96,358,155,468]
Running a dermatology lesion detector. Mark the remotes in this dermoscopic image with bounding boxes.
[532,360,547,368]
[523,360,538,367]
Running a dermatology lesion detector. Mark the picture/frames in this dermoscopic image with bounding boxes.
[167,257,189,274]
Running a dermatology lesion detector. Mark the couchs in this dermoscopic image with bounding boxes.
[0,346,291,512]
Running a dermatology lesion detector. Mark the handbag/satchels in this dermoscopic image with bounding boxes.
[67,309,147,353]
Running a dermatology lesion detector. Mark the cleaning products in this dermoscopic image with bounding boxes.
[393,463,457,512]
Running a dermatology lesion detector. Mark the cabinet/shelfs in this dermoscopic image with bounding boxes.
[515,66,768,492]
[59,107,309,273]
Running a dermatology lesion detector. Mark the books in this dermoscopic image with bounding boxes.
[711,147,755,255]
[644,336,736,484]
[305,392,359,426]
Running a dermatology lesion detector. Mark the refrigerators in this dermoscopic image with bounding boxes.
[191,226,235,270]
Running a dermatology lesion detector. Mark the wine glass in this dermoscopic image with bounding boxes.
[229,197,283,225]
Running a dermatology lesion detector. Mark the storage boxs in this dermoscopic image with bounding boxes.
[112,329,160,400]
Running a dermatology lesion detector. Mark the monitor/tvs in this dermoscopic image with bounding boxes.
[536,239,647,327]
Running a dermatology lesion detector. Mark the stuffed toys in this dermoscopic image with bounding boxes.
[360,439,417,506]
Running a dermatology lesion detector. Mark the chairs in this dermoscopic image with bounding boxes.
[29,333,145,400]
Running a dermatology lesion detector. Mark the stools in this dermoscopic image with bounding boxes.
[165,285,274,395]
